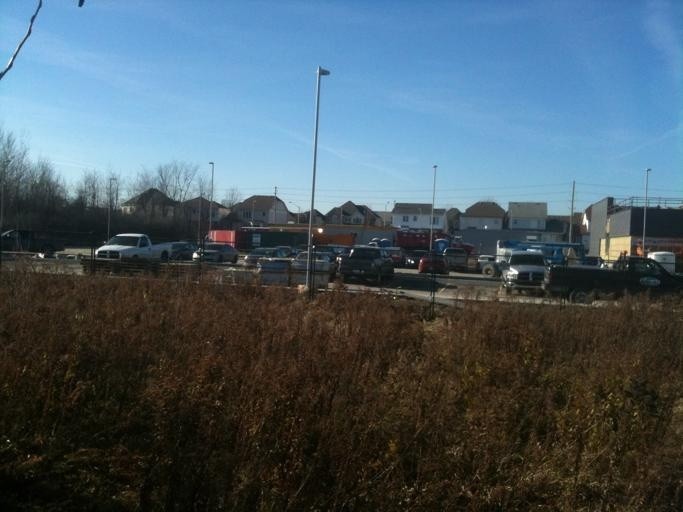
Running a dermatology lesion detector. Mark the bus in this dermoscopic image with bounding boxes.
[649,251,676,274]
[497,239,585,264]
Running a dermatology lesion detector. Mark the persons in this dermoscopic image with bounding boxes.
[636,241,643,256]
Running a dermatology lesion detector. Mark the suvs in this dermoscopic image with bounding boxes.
[500,250,547,294]
[476,255,495,272]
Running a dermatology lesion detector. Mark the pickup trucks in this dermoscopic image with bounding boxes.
[336,243,394,284]
[544,257,681,304]
[0,229,64,257]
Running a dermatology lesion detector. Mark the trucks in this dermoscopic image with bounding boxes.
[395,229,474,251]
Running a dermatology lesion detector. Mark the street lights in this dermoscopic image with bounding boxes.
[429,166,437,251]
[106,177,117,241]
[305,64,330,281]
[642,169,652,256]
[208,161,214,230]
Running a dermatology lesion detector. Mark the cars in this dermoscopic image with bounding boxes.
[386,246,466,273]
[94,232,152,259]
[245,238,350,285]
[155,240,238,263]
